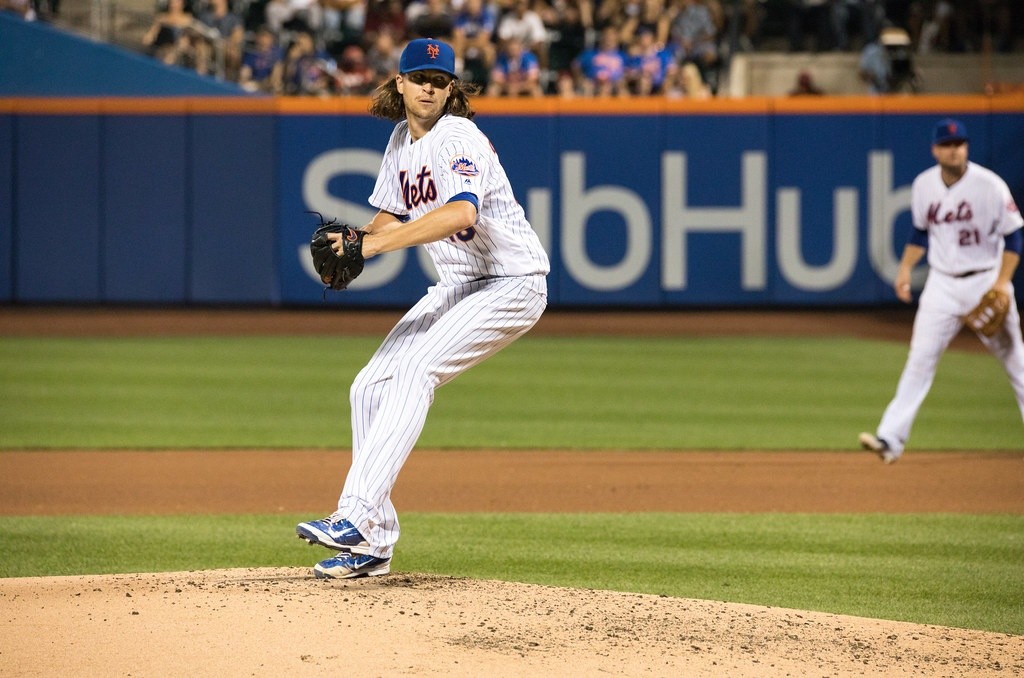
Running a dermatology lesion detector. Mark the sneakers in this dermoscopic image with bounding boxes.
[296,508,371,556]
[859,432,897,465]
[313,550,393,580]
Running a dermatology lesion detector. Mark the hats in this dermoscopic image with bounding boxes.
[932,118,969,146]
[399,37,460,81]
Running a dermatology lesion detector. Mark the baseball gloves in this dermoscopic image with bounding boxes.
[302,209,374,302]
[960,287,1011,339]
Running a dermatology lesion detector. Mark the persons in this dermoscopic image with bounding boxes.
[851,30,914,94]
[24,0,60,24]
[859,123,1024,462]
[144,0,1024,99]
[296,38,551,579]
